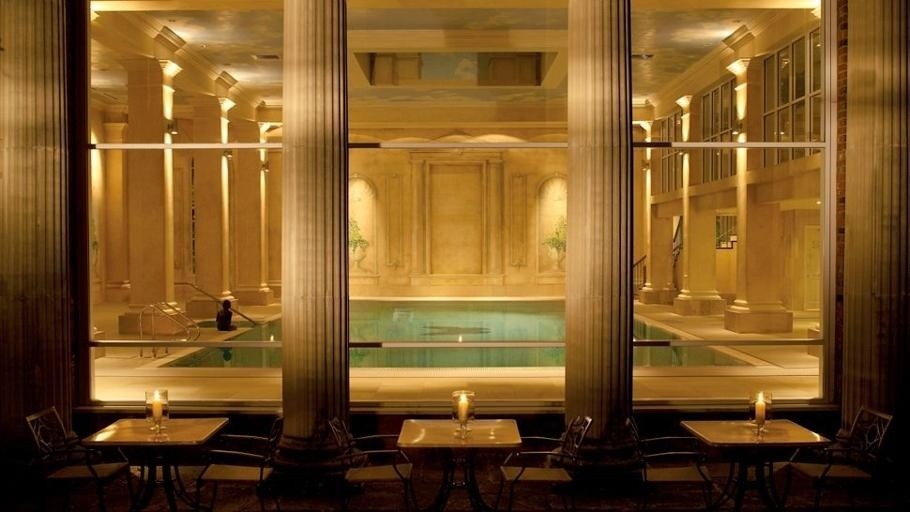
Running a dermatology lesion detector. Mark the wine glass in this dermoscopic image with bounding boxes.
[450,388,478,440]
[747,389,773,437]
[144,388,171,443]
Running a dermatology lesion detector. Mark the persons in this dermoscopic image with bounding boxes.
[220,347,231,361]
[216,300,237,331]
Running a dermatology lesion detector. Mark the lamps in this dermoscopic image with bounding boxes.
[730,118,745,137]
[223,148,233,158]
[261,158,271,175]
[639,159,651,172]
[165,120,179,137]
